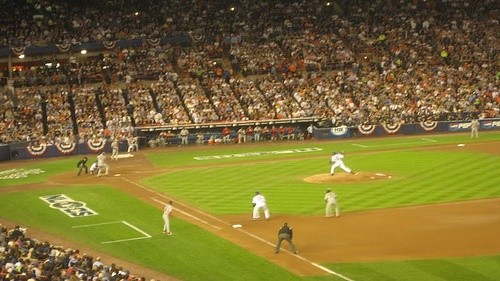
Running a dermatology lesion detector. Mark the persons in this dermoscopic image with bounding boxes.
[90,160,97,175]
[252,192,270,219]
[317,0,500,138]
[330,151,356,176]
[1,0,134,157]
[0,224,160,281]
[77,158,88,176]
[163,201,173,235]
[274,223,299,254]
[97,152,109,177]
[134,0,317,147]
[324,189,339,217]
